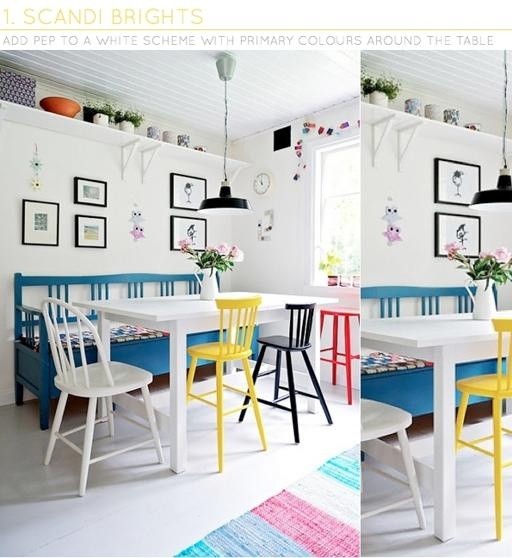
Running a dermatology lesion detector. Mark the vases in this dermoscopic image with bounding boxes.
[199,267,219,300]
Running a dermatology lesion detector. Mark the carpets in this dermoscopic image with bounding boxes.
[171,443,360,557]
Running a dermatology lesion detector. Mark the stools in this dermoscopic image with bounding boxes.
[320,309,360,405]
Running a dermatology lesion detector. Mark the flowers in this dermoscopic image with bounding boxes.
[445,241,512,292]
[177,239,244,278]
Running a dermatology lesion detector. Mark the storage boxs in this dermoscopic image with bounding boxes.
[0,67,37,107]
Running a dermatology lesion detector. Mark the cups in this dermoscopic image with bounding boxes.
[327,275,337,287]
[147,126,207,152]
[353,277,362,288]
[339,275,351,287]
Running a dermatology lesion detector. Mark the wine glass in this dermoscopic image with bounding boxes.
[453,176,462,197]
[184,187,192,204]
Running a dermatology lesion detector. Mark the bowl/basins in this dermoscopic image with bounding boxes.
[40,96,82,118]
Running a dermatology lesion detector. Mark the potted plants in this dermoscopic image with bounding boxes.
[361,70,402,108]
[319,251,345,286]
[86,99,116,127]
[113,108,145,133]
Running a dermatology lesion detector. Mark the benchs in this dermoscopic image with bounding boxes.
[13,272,259,430]
[360,286,506,462]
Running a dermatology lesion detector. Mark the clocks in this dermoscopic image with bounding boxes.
[252,171,272,196]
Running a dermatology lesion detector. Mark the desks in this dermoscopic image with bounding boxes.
[70,293,339,474]
[360,309,512,542]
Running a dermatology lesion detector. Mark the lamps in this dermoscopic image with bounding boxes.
[469,51,512,209]
[198,52,250,212]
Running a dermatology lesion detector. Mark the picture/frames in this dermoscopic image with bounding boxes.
[434,158,481,206]
[434,212,481,258]
[19,172,208,252]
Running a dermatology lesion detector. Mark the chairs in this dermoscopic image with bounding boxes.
[361,398,426,531]
[456,318,512,542]
[186,297,267,472]
[39,298,165,498]
[238,303,334,443]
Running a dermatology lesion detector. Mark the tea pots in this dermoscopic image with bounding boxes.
[193,267,220,302]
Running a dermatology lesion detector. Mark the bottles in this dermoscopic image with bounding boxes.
[404,98,460,126]
